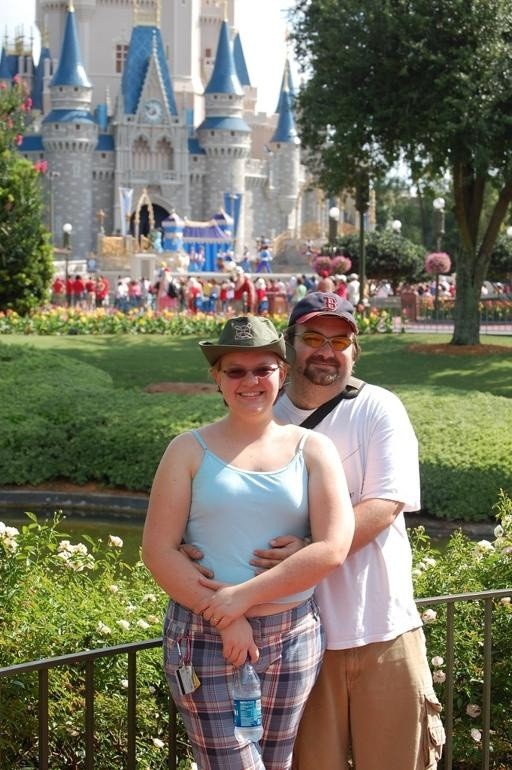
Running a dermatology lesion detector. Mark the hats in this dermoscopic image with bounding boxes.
[199,316,286,367]
[288,292,359,335]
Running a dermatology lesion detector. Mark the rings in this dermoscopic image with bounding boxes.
[213,616,220,622]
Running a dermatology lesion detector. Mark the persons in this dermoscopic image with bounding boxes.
[480,280,511,295]
[141,317,354,769]
[190,246,205,271]
[52,273,111,311]
[368,277,456,297]
[241,246,252,273]
[114,274,320,317]
[216,249,233,269]
[178,292,445,769]
[256,246,273,273]
[330,272,360,308]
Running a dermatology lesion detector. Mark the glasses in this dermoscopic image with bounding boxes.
[295,332,355,352]
[217,365,280,380]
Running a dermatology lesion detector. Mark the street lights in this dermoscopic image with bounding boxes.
[62,223,74,281]
[353,171,370,321]
[327,205,342,241]
[390,219,403,236]
[48,170,62,245]
[431,196,446,323]
